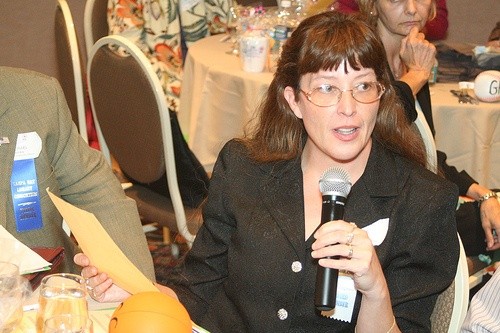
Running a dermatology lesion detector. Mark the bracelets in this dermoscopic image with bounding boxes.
[476,192,498,207]
[355,316,395,333]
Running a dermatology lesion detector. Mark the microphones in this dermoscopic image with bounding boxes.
[314,167,353,311]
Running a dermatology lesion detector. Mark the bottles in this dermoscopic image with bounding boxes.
[428,58,439,97]
[272,1,291,62]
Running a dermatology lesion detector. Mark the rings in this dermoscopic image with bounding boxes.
[350,223,357,232]
[347,233,354,245]
[93,292,96,297]
[87,286,93,290]
[348,247,353,258]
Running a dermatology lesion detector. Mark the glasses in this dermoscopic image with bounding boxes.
[299,81,384,107]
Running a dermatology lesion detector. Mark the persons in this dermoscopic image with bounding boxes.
[74,11,460,333]
[0,64,157,283]
[106,0,237,208]
[337,0,500,269]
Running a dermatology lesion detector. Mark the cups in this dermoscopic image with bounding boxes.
[239,36,269,75]
[36,273,89,333]
[0,262,24,333]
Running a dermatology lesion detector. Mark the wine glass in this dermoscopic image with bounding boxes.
[226,7,251,58]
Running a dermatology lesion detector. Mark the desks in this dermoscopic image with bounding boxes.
[172,27,500,192]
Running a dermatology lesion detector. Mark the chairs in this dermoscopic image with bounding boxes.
[53,0,468,333]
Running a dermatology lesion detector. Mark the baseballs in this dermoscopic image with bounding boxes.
[473,70,500,102]
